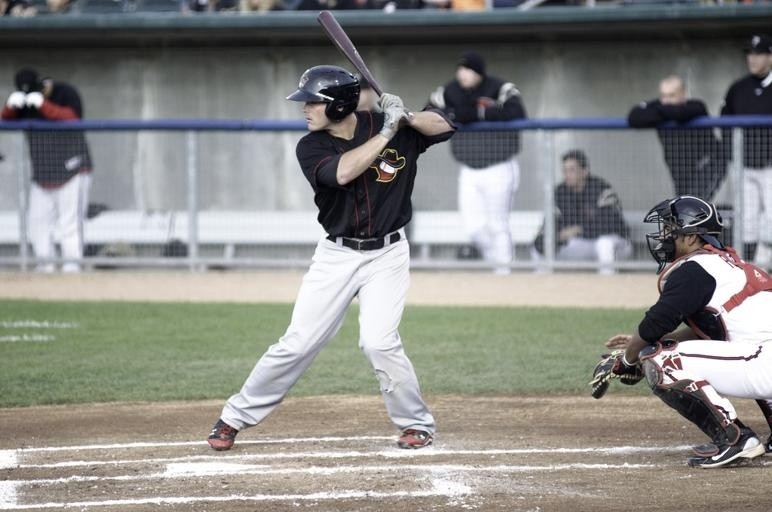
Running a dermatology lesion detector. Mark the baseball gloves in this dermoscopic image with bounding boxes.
[592,349,645,398]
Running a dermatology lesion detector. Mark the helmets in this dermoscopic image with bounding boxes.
[644,195,723,273]
[285,65,360,124]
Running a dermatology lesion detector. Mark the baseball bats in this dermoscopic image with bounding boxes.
[317,10,383,96]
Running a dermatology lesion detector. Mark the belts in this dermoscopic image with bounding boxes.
[325,231,401,251]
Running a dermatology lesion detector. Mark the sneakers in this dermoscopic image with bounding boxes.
[689,426,766,470]
[397,428,434,449]
[207,420,239,452]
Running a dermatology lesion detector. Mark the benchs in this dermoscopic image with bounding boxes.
[0,211,173,256]
[623,210,666,245]
[176,210,545,259]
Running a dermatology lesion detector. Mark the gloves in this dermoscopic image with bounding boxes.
[376,91,415,141]
[26,91,45,109]
[6,92,26,109]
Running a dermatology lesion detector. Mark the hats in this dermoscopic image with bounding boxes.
[741,30,770,56]
[15,68,45,95]
[457,53,488,75]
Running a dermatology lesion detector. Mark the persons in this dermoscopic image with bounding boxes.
[719,32,771,265]
[530,149,633,280]
[205,64,459,452]
[351,71,379,113]
[1,65,94,273]
[627,72,727,204]
[425,51,527,273]
[589,191,771,470]
